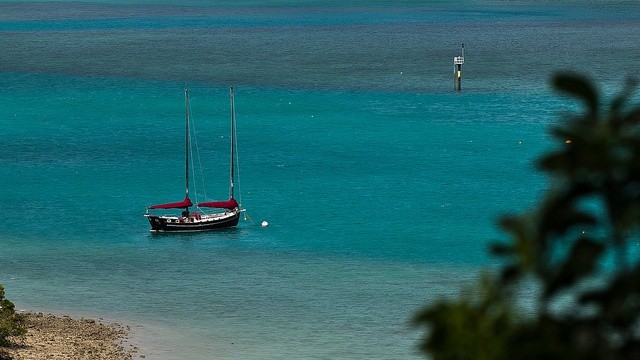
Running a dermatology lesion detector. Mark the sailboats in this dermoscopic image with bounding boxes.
[144,86,246,233]
